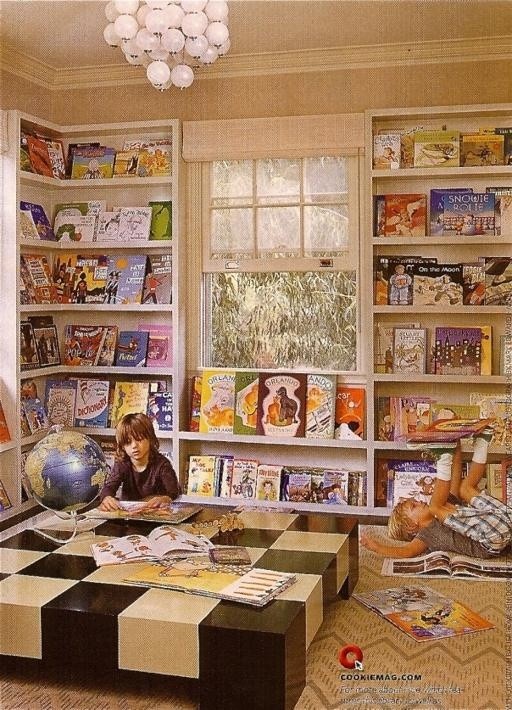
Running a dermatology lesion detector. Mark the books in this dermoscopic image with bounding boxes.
[15,129,512,510]
[380,551,512,582]
[352,583,497,643]
[0,479,13,512]
[89,525,215,568]
[78,501,205,524]
[121,559,299,607]
[209,547,252,566]
[0,400,11,446]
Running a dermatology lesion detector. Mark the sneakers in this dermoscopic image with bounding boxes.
[461,428,495,445]
[417,442,457,462]
[391,301,398,305]
[400,301,408,305]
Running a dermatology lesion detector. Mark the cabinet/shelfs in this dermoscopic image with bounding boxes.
[369,99,511,520]
[0,111,187,532]
[178,430,369,519]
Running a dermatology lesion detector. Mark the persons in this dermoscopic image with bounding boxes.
[359,426,512,558]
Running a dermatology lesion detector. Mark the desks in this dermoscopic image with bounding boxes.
[0,497,363,710]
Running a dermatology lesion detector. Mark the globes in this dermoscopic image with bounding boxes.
[25,430,109,543]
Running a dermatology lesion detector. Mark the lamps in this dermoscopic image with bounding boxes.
[102,0,232,94]
